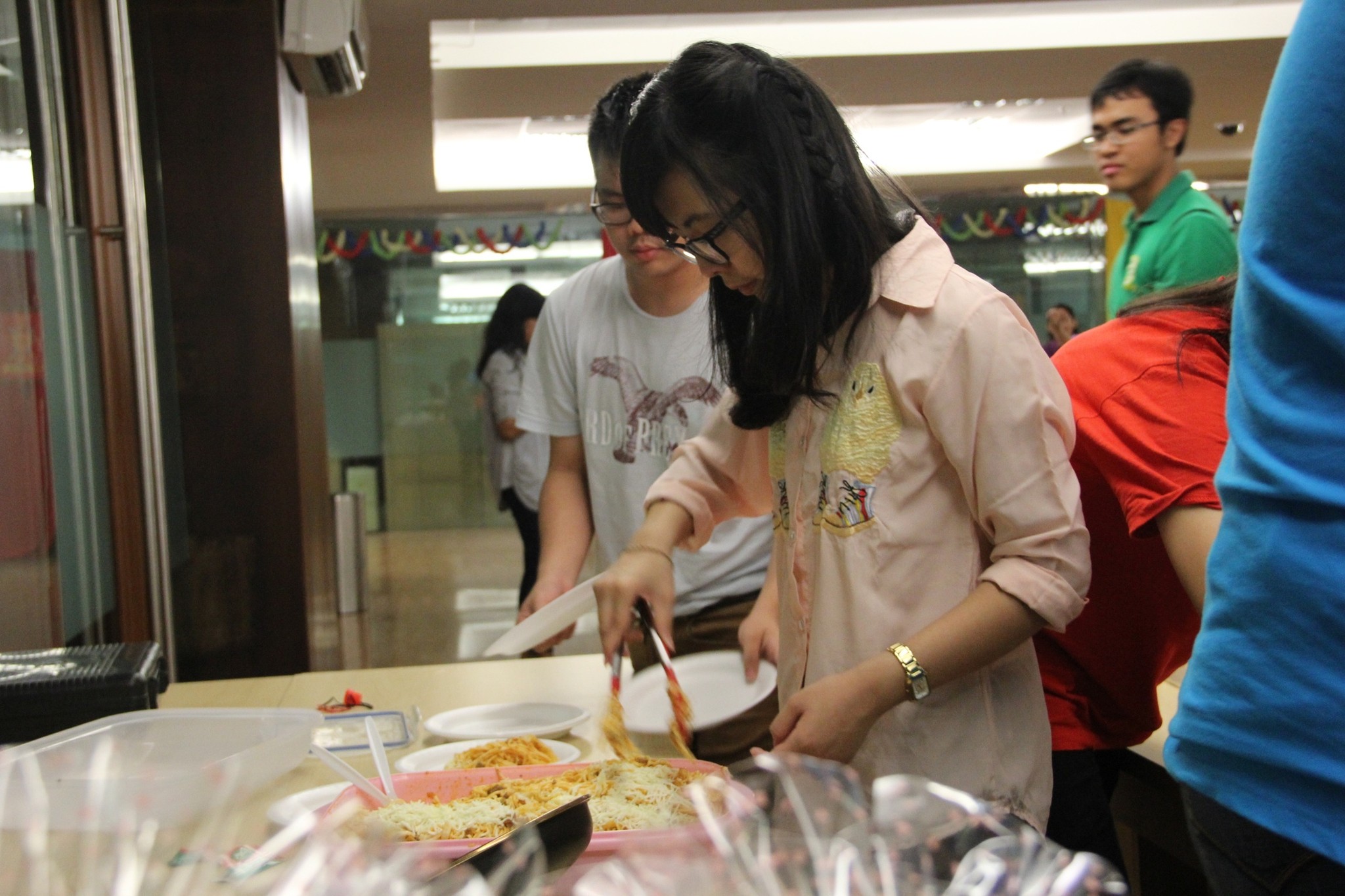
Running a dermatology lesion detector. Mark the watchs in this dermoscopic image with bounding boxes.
[887,642,931,701]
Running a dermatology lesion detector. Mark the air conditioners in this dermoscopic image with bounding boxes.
[282,0,375,101]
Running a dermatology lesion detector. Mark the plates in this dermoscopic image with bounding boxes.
[480,568,612,657]
[622,650,777,732]
[394,701,590,773]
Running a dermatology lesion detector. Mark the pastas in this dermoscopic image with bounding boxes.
[347,684,721,841]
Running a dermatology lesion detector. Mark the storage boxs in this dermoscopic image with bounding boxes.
[0,708,325,833]
[0,639,171,744]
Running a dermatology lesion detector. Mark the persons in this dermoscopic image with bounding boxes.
[475,283,553,661]
[515,70,783,784]
[620,40,1091,834]
[1044,302,1080,355]
[1031,272,1242,896]
[1090,59,1239,318]
[1165,0,1345,896]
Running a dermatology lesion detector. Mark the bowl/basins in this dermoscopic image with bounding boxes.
[0,708,325,830]
[323,756,741,877]
[412,792,593,896]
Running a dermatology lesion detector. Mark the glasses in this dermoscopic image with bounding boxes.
[590,181,637,225]
[1081,120,1166,151]
[663,196,749,264]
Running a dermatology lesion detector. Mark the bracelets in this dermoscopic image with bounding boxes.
[624,546,676,564]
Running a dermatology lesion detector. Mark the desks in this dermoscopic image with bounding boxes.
[149,651,633,896]
